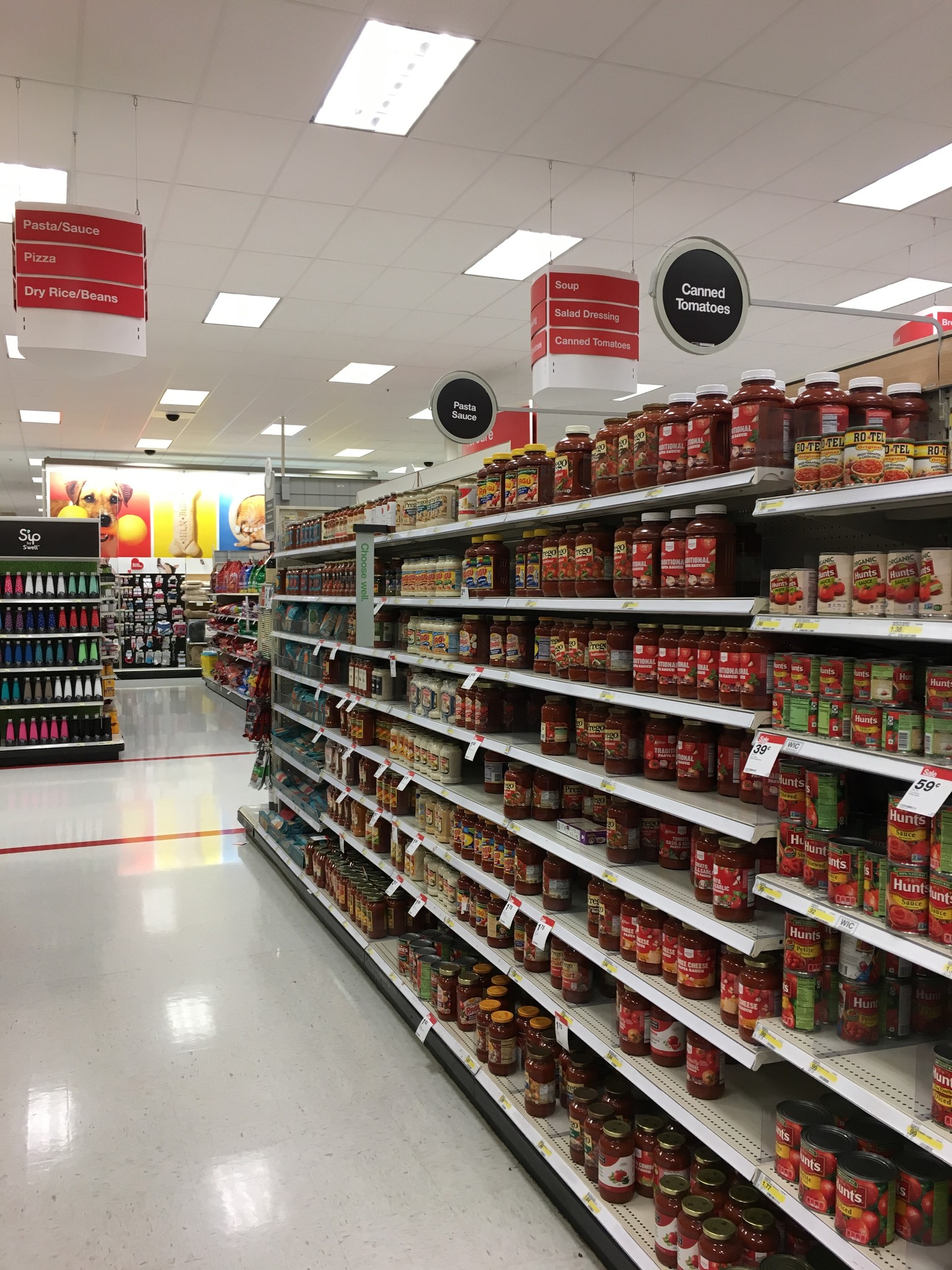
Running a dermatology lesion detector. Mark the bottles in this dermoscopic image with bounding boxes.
[278,482,401,599]
[2,567,115,746]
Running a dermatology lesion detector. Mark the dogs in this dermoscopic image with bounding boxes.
[65,479,133,559]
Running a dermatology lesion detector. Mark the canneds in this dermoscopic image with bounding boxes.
[278,420,951,1270]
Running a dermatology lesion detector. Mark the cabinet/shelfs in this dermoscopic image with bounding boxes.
[185,600,211,677]
[0,597,126,756]
[745,474,952,1270]
[270,466,797,1270]
[202,591,266,710]
